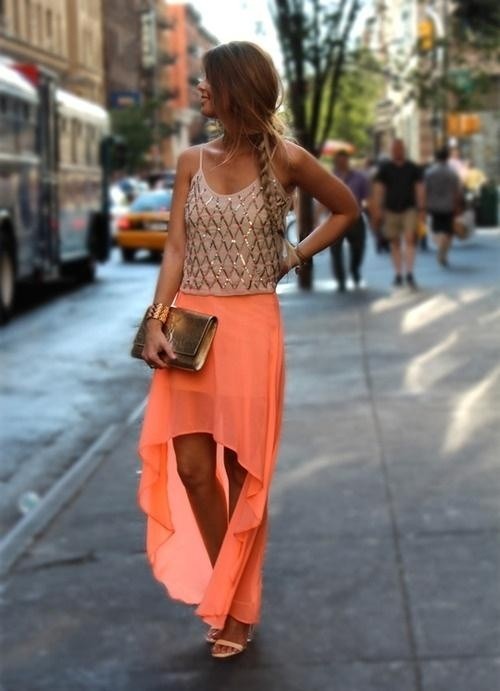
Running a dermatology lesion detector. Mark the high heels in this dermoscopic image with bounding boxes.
[205,627,223,644]
[211,624,254,657]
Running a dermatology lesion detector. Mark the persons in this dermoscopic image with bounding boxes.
[367,138,425,296]
[312,146,368,293]
[419,143,464,269]
[131,38,367,662]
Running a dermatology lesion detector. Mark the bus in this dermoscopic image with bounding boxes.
[0,54,118,322]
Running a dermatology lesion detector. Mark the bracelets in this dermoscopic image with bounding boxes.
[292,247,309,274]
[144,302,169,325]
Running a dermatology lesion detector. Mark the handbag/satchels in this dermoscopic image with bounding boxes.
[131,305,218,373]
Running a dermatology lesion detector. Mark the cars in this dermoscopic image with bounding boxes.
[103,167,177,262]
